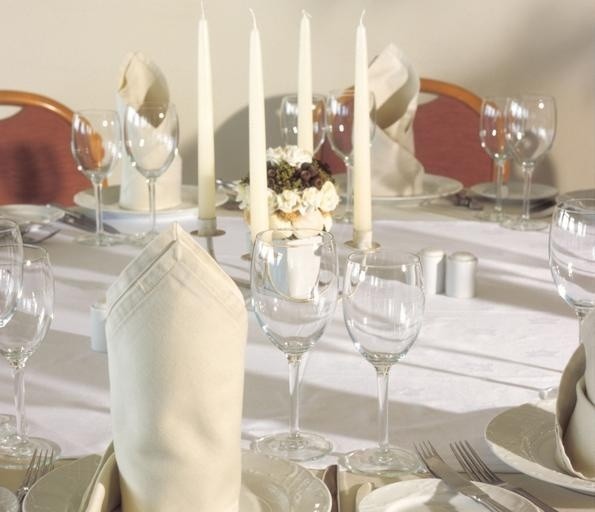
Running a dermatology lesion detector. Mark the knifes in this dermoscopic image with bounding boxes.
[423,458,512,512]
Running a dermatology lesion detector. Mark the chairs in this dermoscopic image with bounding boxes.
[322,75,509,186]
[0,91,108,209]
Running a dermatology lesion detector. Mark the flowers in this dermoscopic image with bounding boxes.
[228,144,340,245]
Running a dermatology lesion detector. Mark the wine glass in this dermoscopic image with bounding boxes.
[72,109,132,247]
[1,218,61,469]
[280,88,377,226]
[343,250,424,473]
[251,228,339,462]
[477,95,557,231]
[540,197,595,401]
[124,107,178,247]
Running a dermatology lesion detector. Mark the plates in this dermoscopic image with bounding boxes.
[485,399,595,493]
[470,181,558,205]
[325,172,466,207]
[0,204,66,226]
[22,449,333,512]
[74,183,231,218]
[359,477,544,512]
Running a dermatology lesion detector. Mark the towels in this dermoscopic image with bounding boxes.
[367,41,426,197]
[550,306,595,483]
[114,50,183,211]
[104,219,248,512]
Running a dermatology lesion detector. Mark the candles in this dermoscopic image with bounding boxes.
[248,9,270,243]
[352,8,371,247]
[197,0,217,237]
[297,9,314,167]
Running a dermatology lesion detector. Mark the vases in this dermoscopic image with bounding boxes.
[285,241,322,299]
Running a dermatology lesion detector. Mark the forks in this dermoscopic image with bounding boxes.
[16,448,54,504]
[411,440,560,512]
